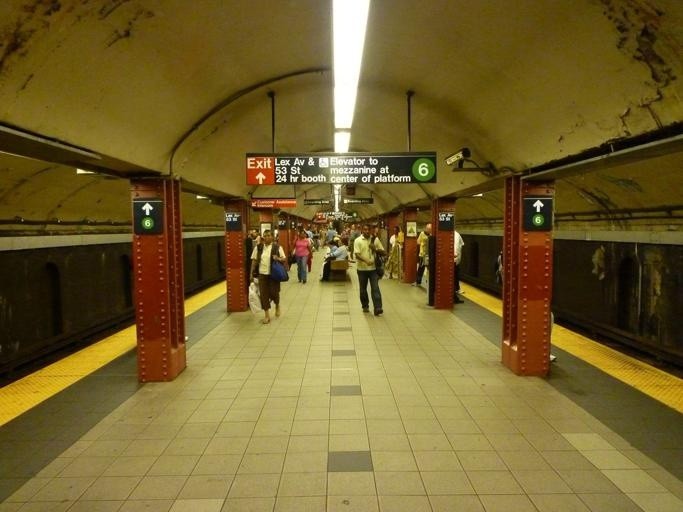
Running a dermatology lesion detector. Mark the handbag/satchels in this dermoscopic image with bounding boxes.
[288,255,296,263]
[272,261,288,282]
[375,256,385,277]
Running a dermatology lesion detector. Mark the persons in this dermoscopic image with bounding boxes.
[246,222,465,305]
[352,224,387,316]
[495,249,503,298]
[247,228,287,325]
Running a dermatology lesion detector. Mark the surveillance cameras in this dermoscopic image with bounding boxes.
[445,147,470,168]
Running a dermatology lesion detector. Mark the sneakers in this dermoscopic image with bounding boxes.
[455,299,463,304]
[374,307,383,315]
[263,308,280,324]
[363,307,369,312]
[299,280,306,283]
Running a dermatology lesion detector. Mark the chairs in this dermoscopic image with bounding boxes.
[331,255,348,280]
